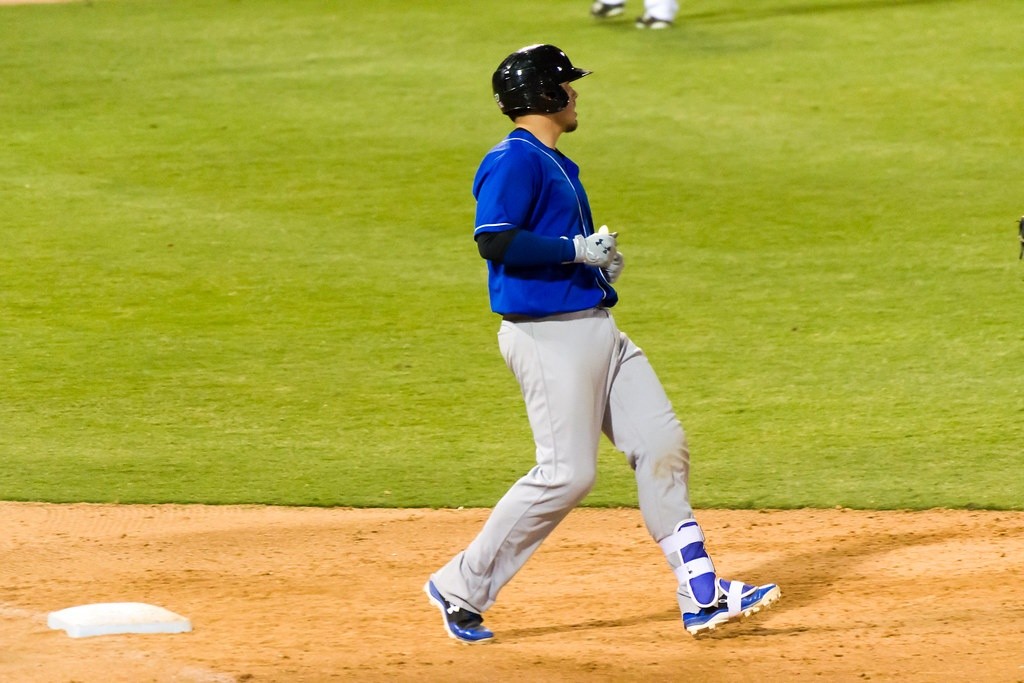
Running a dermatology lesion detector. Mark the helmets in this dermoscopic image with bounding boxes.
[492,44,593,115]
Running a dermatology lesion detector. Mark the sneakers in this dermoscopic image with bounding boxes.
[591,2,624,17]
[424,580,495,645]
[636,16,671,30]
[683,584,781,637]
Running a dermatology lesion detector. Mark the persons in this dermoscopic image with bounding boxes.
[423,43,781,645]
[589,0,679,29]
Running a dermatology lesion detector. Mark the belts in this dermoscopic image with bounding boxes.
[503,313,537,321]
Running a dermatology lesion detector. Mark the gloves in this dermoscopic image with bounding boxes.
[607,251,625,283]
[572,224,619,269]
